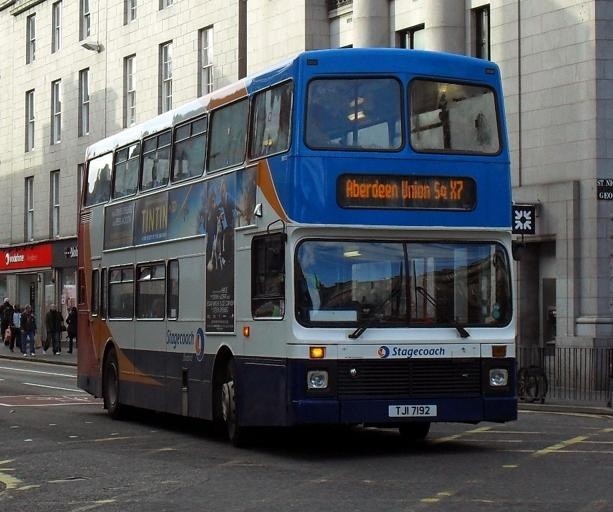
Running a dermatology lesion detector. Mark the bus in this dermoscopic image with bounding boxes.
[73,45,519,446]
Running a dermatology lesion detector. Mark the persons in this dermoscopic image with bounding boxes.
[0,298,77,357]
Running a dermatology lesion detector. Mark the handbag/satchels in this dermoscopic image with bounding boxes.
[4,328,12,346]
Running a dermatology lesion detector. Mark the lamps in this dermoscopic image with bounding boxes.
[80,42,97,52]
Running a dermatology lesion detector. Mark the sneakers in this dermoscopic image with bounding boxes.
[23,350,72,357]
